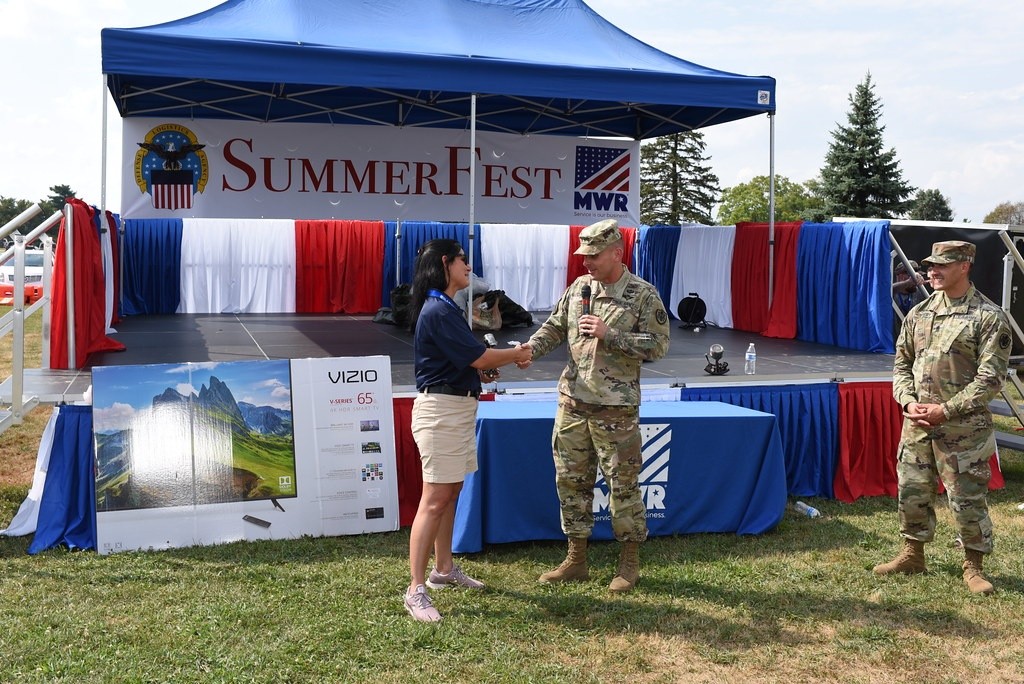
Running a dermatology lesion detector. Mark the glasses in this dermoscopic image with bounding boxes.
[456,253,468,266]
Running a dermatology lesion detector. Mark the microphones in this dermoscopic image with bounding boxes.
[581,285,590,336]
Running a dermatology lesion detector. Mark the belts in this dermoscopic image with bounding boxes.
[419,386,480,399]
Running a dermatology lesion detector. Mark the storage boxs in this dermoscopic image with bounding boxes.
[91,356,400,557]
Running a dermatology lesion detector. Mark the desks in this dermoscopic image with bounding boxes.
[452,398,788,555]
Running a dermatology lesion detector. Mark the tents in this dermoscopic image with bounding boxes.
[100,0,776,335]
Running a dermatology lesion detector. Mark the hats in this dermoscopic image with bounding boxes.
[895,260,926,276]
[573,219,622,255]
[921,240,976,266]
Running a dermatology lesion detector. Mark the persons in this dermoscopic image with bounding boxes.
[517,219,670,594]
[893,259,924,350]
[403,239,533,624]
[873,241,1011,594]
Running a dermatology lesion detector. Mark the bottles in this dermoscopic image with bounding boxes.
[745,343,756,375]
[794,501,820,519]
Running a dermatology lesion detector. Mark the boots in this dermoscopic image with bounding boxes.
[962,554,993,595]
[539,537,588,585]
[609,541,639,593]
[873,539,925,576]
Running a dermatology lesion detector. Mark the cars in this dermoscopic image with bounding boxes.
[0,249,55,289]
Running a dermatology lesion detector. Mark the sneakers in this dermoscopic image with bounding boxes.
[403,584,442,623]
[426,562,485,592]
